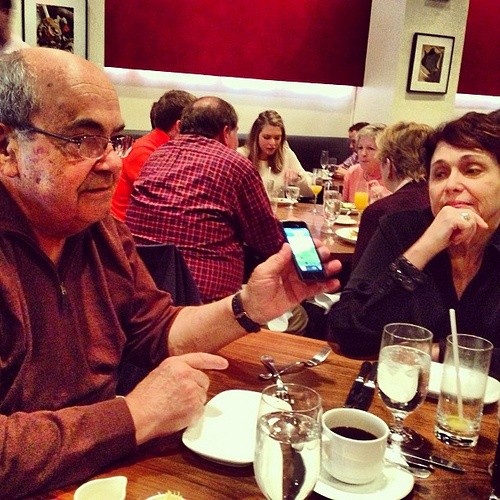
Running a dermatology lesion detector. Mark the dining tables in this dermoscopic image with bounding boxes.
[270,201,366,255]
[37,327,500,500]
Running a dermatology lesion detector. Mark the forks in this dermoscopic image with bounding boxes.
[260,354,288,400]
[258,347,331,380]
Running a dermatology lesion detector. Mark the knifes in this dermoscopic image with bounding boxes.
[400,447,465,472]
[342,361,371,408]
[355,362,378,411]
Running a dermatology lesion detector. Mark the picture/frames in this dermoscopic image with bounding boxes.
[407,32,456,95]
[21,0,88,60]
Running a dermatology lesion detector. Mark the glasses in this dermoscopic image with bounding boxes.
[11,122,137,160]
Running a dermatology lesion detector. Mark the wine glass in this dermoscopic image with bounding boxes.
[354,181,368,227]
[376,323,433,451]
[321,151,337,183]
[253,383,321,500]
[286,171,300,211]
[310,168,322,212]
[323,182,339,205]
[322,193,340,234]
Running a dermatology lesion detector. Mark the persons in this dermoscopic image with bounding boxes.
[330,122,370,179]
[125,96,309,337]
[342,123,394,206]
[236,110,315,199]
[111,90,197,223]
[0,0,31,55]
[351,121,435,274]
[325,108,500,382]
[0,46,342,500]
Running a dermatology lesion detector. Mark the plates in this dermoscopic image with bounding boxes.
[270,198,298,205]
[339,203,359,214]
[182,390,293,467]
[335,227,359,244]
[325,215,357,224]
[302,439,415,500]
[427,362,500,405]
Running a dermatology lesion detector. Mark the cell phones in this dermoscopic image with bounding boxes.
[281,221,325,280]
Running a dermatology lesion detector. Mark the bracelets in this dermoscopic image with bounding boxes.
[231,292,261,334]
[389,254,426,292]
[295,173,303,184]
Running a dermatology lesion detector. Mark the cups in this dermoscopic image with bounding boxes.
[434,334,493,447]
[321,408,390,484]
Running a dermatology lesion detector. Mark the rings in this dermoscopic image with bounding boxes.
[462,211,470,221]
